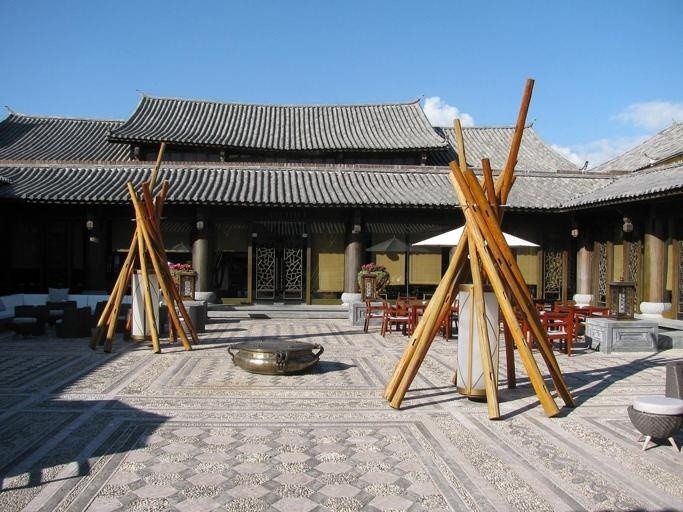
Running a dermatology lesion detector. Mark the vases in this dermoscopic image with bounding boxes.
[355,270,390,303]
[170,270,197,301]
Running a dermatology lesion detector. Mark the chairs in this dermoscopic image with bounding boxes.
[362,290,610,355]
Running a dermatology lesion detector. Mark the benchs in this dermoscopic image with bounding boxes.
[666,361,682,400]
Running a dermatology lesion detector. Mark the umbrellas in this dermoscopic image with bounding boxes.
[411,226,541,248]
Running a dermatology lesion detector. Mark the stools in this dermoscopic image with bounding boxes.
[627,393,680,453]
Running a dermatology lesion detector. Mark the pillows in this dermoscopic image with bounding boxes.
[48,288,69,302]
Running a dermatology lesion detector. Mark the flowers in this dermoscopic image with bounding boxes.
[361,261,389,277]
[169,261,195,274]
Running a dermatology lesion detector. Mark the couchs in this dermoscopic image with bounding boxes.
[0,292,167,341]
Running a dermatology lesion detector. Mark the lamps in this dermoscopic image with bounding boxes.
[131,268,159,342]
[455,284,500,400]
